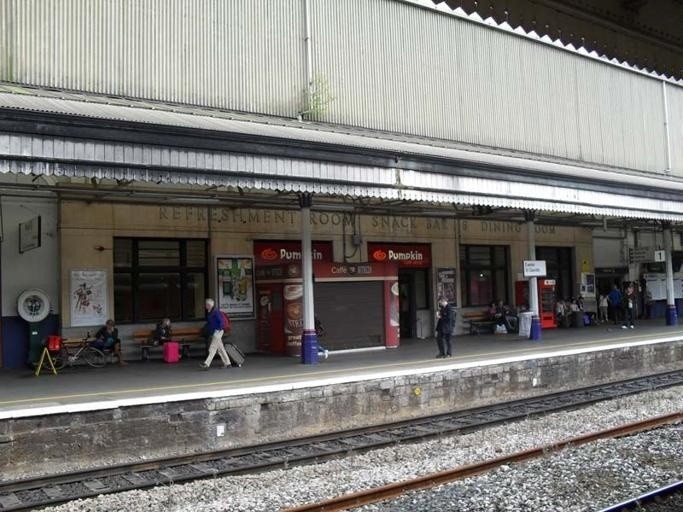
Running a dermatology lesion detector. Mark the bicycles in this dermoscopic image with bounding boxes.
[41,330,108,369]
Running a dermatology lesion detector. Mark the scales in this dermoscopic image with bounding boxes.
[15,288,54,369]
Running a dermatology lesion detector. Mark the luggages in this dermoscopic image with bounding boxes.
[224,342,245,367]
[164,342,180,363]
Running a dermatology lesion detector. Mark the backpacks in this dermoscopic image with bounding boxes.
[220,311,231,334]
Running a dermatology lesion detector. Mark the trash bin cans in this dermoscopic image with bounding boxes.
[517,312,535,338]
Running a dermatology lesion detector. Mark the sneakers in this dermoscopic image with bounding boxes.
[221,365,232,368]
[620,325,635,329]
[199,362,208,371]
[323,349,329,360]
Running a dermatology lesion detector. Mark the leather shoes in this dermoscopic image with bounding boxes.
[435,353,452,359]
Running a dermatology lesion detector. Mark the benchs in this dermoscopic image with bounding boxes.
[60,336,120,364]
[465,310,515,335]
[132,327,228,361]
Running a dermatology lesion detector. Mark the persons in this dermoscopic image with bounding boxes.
[86,319,119,357]
[555,284,636,330]
[487,300,532,331]
[152,296,231,371]
[435,296,456,361]
